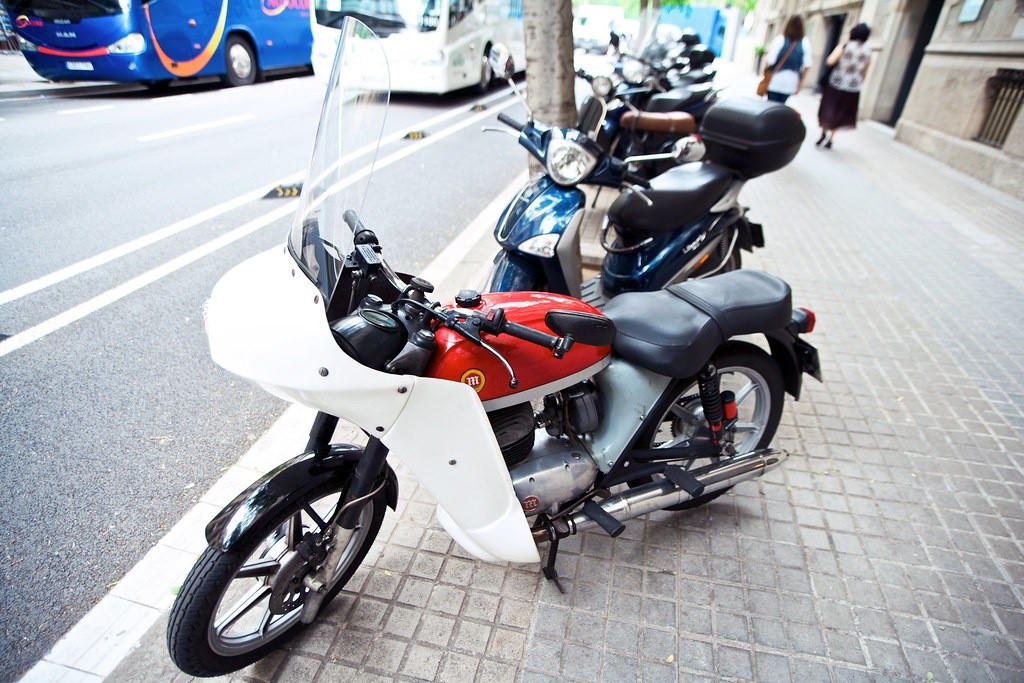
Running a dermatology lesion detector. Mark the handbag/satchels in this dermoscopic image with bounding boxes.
[818,63,833,88]
[755,65,773,97]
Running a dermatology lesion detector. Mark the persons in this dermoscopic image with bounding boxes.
[756,15,809,109]
[810,24,874,149]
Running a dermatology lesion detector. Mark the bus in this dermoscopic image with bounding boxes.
[337,0,526,99]
[0,0,316,91]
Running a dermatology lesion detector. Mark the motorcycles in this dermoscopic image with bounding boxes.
[165,13,825,681]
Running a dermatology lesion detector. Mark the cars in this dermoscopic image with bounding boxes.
[568,6,625,56]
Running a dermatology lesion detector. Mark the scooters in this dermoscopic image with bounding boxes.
[606,23,717,113]
[570,67,728,208]
[481,96,809,323]
